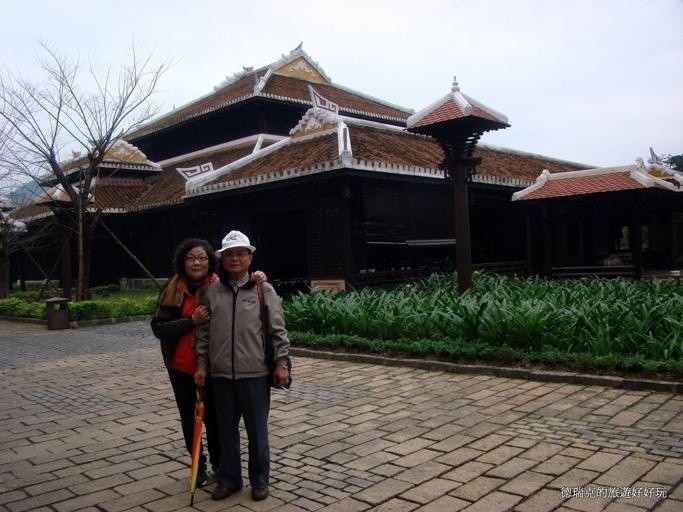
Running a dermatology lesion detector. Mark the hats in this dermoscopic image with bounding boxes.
[213,230,256,258]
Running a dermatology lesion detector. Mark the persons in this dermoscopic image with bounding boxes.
[149,237,269,487]
[193,229,292,500]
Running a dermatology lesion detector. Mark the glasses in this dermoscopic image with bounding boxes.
[184,253,208,262]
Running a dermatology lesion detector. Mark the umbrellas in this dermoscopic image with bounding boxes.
[189,378,206,508]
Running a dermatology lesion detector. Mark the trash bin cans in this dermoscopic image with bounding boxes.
[44,297,70,330]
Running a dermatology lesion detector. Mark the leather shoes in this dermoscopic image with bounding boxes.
[251,487,268,500]
[211,484,242,500]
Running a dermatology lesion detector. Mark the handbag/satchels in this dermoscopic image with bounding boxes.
[257,282,291,390]
